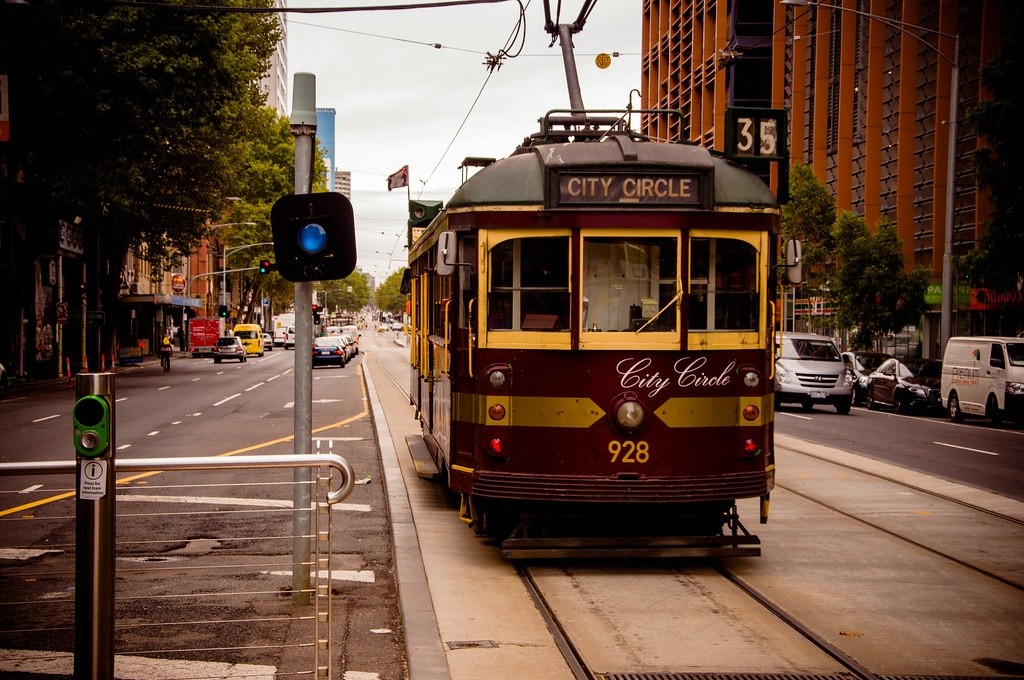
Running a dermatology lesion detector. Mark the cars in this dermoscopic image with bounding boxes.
[354,315,404,332]
[312,335,356,368]
[841,351,943,414]
[263,333,272,351]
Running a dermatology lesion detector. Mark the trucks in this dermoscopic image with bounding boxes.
[274,314,295,346]
[188,317,218,358]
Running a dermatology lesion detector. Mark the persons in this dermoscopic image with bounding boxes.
[161,332,172,372]
[176,325,185,351]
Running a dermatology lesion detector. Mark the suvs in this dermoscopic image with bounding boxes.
[214,336,248,363]
[283,326,295,350]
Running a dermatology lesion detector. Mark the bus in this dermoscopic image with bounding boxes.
[398,109,803,558]
[320,312,354,327]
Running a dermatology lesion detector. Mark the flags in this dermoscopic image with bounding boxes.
[386,165,408,191]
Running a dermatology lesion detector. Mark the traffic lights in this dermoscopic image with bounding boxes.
[312,305,317,315]
[260,260,271,274]
[271,192,357,282]
[314,314,320,325]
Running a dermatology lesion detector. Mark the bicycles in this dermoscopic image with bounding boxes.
[160,353,173,375]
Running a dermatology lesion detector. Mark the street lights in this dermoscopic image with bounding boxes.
[779,0,960,361]
[206,222,257,316]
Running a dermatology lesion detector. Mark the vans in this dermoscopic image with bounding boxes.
[327,326,357,335]
[772,332,853,415]
[939,336,1024,428]
[233,324,264,357]
